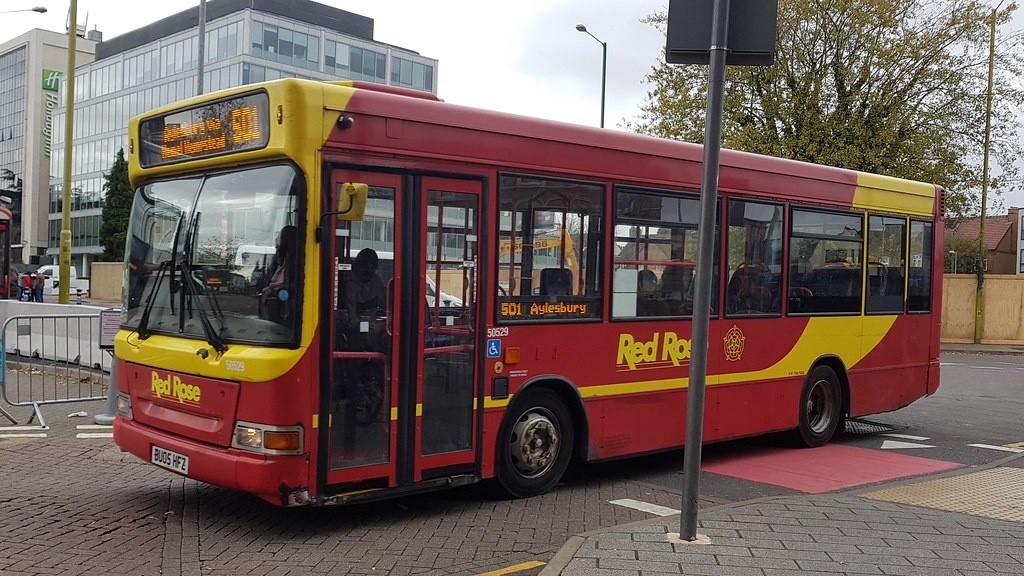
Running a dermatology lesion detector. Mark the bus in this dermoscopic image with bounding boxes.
[114,77,943,504]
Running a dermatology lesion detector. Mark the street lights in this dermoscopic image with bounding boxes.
[575,25,606,128]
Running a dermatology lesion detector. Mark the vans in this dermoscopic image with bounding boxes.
[29,265,76,280]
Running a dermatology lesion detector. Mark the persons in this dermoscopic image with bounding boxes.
[17,271,29,301]
[32,276,37,302]
[22,272,33,288]
[343,247,388,332]
[248,225,297,299]
[34,271,44,303]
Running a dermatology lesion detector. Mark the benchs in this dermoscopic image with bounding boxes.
[333,261,459,350]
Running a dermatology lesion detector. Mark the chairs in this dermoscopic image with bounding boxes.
[637,261,932,318]
